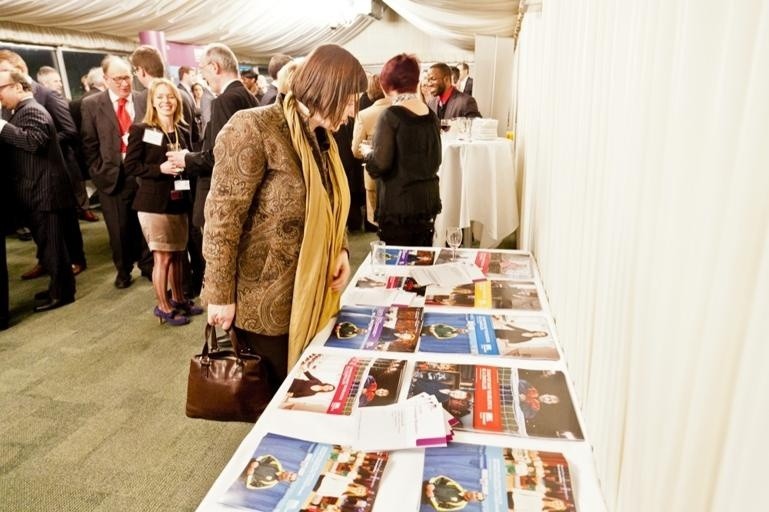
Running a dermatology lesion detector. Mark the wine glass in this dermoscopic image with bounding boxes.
[441,117,452,145]
[446,226,464,262]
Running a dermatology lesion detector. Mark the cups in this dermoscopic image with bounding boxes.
[369,240,385,275]
[166,144,182,173]
[451,115,472,143]
[360,140,373,165]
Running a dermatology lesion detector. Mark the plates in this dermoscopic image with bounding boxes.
[473,119,497,140]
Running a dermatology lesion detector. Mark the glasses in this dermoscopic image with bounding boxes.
[108,74,130,83]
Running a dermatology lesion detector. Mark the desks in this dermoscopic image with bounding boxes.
[195,246,610,512]
[432,137,520,250]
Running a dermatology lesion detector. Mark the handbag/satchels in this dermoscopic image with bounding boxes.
[186,352,270,423]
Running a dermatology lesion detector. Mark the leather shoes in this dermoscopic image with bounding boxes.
[84,209,98,220]
[17,227,32,240]
[116,274,130,287]
[22,263,86,311]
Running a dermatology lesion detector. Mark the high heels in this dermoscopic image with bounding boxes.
[154,298,203,326]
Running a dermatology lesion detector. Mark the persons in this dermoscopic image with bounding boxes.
[0,45,259,330]
[166,43,484,396]
[237,255,575,512]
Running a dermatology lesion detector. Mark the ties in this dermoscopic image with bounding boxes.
[117,99,132,152]
